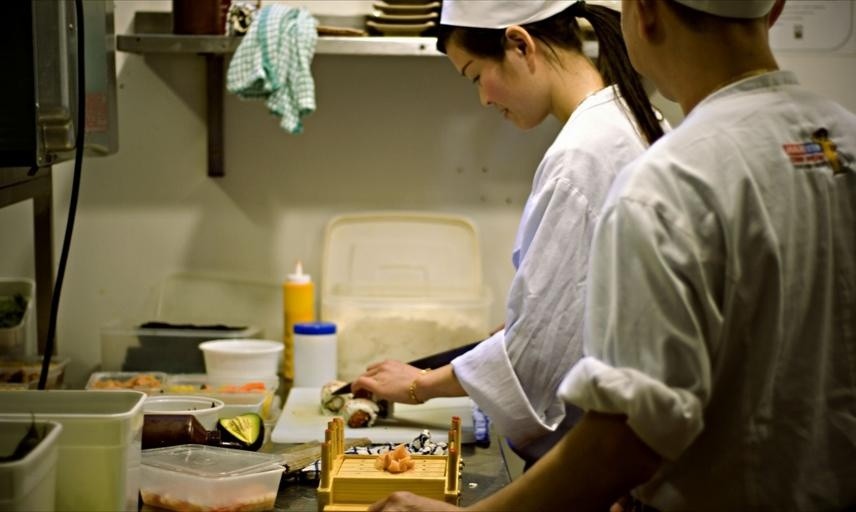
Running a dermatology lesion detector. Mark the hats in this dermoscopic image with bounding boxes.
[439,0,579,30]
[678,0,777,18]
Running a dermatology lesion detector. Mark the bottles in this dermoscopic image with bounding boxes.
[282,260,315,381]
[293,322,338,387]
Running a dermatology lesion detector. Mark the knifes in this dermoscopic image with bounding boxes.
[332,336,490,396]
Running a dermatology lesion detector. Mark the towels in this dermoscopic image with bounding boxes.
[224,2,318,136]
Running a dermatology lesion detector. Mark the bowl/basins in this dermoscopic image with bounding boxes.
[142,395,224,431]
[198,338,285,376]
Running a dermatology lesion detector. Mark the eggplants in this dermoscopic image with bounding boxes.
[217,414,266,452]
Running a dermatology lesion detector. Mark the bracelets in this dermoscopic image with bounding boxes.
[410,367,432,404]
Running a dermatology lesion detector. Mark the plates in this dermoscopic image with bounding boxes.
[364,1,440,35]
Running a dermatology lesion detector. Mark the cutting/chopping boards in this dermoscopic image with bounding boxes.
[270,386,477,443]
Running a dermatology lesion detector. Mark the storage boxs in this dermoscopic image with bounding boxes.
[319,215,492,385]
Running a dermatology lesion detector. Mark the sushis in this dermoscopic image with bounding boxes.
[320,380,394,429]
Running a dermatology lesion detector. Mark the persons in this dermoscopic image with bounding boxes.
[349,0,677,465]
[368,1,856,512]
